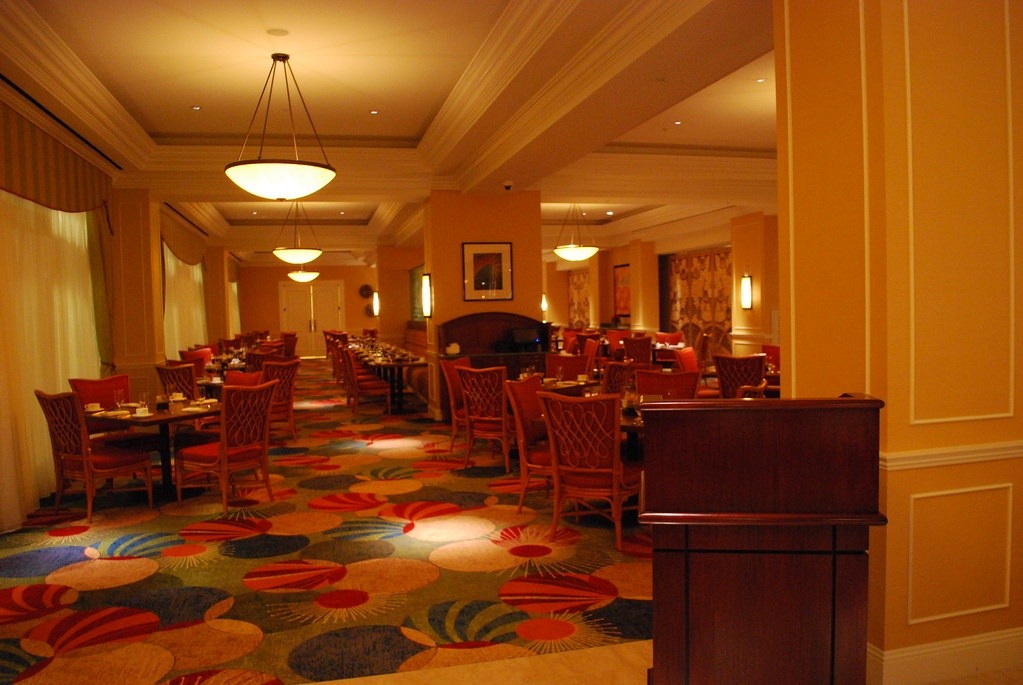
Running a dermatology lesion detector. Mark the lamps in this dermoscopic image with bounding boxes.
[223,54,337,200]
[273,199,323,265]
[287,265,319,283]
[740,276,752,310]
[422,273,432,318]
[542,293,547,312]
[553,203,599,262]
[372,291,379,316]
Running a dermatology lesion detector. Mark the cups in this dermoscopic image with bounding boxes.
[84,404,100,410]
[136,408,148,415]
[578,375,589,381]
[170,393,183,399]
[528,365,537,375]
[212,377,221,382]
[166,384,176,398]
[521,368,530,380]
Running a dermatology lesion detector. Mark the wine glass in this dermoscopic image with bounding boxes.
[113,389,125,412]
[633,394,648,424]
[138,392,150,408]
[196,386,206,409]
[555,366,564,381]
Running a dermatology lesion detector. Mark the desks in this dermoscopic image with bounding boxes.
[196,376,226,400]
[86,392,224,509]
[210,353,246,363]
[367,352,428,415]
[506,378,598,398]
[360,351,420,404]
[205,362,246,381]
[618,394,659,465]
[358,347,414,382]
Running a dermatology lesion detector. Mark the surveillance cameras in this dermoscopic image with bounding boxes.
[504,183,512,190]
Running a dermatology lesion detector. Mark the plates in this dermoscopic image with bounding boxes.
[84,408,104,412]
[132,414,153,417]
[210,381,223,384]
[169,398,187,401]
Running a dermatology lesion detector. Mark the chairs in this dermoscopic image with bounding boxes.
[33,324,781,550]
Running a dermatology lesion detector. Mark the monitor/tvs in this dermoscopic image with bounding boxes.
[510,328,540,344]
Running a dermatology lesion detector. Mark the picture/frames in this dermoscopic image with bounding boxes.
[462,242,513,302]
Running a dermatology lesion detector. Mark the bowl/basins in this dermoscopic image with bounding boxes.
[544,378,557,383]
